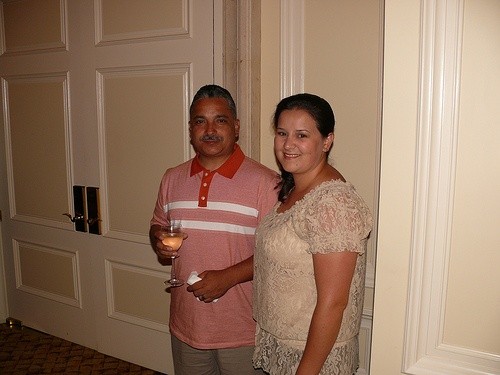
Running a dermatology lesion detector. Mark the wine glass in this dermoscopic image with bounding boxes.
[161,217,183,286]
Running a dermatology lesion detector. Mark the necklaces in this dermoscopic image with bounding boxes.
[289,185,296,196]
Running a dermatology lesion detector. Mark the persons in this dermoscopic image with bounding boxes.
[251,94,372,375]
[150,85,281,374]
